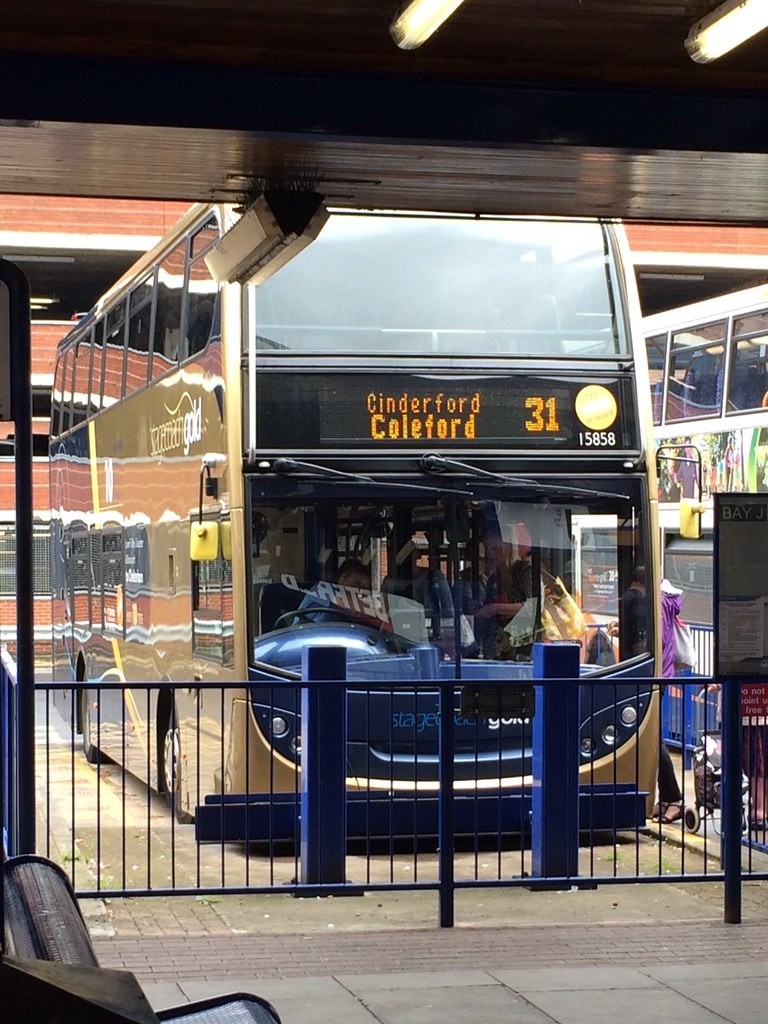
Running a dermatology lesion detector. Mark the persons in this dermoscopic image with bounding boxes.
[621,566,650,660]
[606,579,684,827]
[460,525,544,659]
[333,559,372,612]
[724,436,735,493]
[704,682,768,831]
[381,541,453,620]
[669,437,699,499]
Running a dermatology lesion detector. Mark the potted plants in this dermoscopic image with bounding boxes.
[203,155,331,287]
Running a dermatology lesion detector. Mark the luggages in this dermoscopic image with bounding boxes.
[685,685,749,838]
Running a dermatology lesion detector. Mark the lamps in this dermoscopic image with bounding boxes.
[684,0,768,64]
[389,0,464,50]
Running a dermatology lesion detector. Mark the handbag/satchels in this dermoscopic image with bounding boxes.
[671,615,696,670]
[522,564,589,641]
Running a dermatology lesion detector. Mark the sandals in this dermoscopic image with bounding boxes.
[652,803,686,824]
[651,801,667,818]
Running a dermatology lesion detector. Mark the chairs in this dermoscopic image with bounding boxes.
[258,582,315,633]
[653,352,768,424]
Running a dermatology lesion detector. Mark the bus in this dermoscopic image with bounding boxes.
[53,204,657,845]
[639,286,768,766]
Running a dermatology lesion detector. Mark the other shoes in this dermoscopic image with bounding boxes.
[742,816,768,831]
